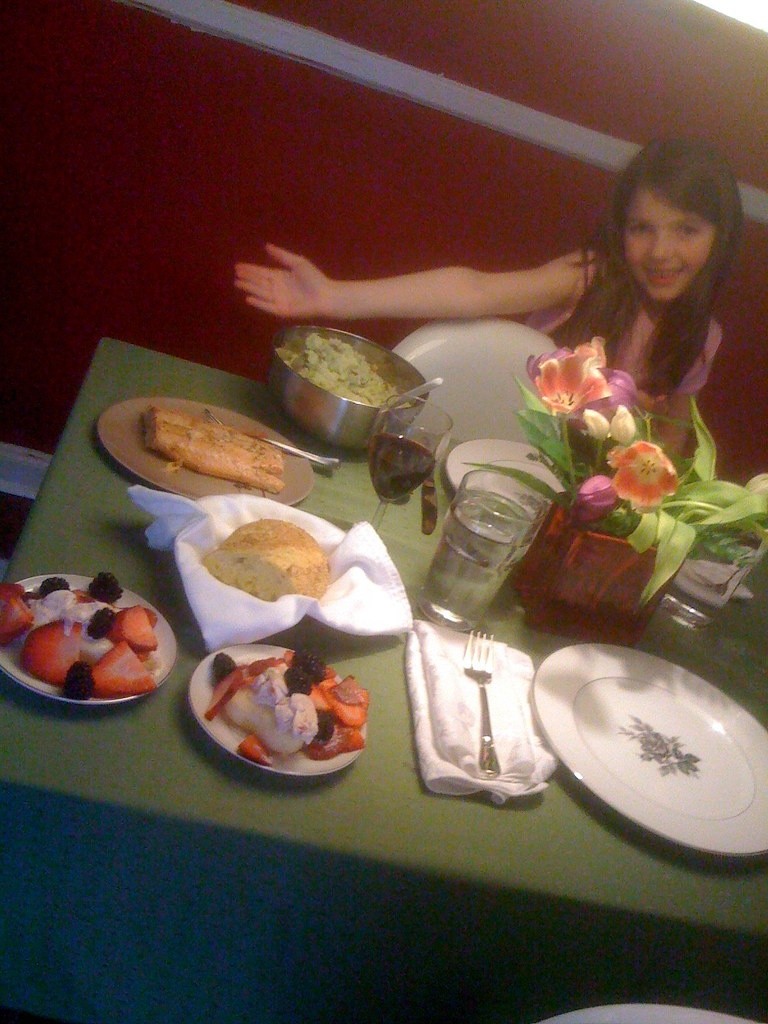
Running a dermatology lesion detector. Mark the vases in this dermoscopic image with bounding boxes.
[514,491,685,642]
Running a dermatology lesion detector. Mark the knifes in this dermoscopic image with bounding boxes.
[421,468,437,535]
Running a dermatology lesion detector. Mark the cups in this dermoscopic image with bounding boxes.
[659,520,768,631]
[417,465,551,631]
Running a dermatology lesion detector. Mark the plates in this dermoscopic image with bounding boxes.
[446,438,568,499]
[187,645,368,776]
[533,642,768,856]
[97,396,315,505]
[0,574,178,705]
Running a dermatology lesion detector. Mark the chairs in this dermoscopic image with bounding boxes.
[390,316,563,446]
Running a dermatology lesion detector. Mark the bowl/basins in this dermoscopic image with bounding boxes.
[270,324,430,451]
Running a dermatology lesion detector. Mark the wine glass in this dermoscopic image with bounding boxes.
[369,393,454,532]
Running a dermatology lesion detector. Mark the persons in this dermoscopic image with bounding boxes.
[232,134,744,456]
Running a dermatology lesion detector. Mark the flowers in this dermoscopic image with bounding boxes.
[455,329,768,616]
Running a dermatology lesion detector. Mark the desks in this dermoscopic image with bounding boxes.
[0,337,768,1024]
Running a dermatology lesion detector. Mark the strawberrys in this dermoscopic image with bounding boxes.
[204,649,369,766]
[0,582,159,698]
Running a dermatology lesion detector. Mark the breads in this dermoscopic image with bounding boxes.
[205,518,331,602]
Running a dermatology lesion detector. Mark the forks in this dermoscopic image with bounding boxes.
[203,406,339,466]
[463,631,502,778]
